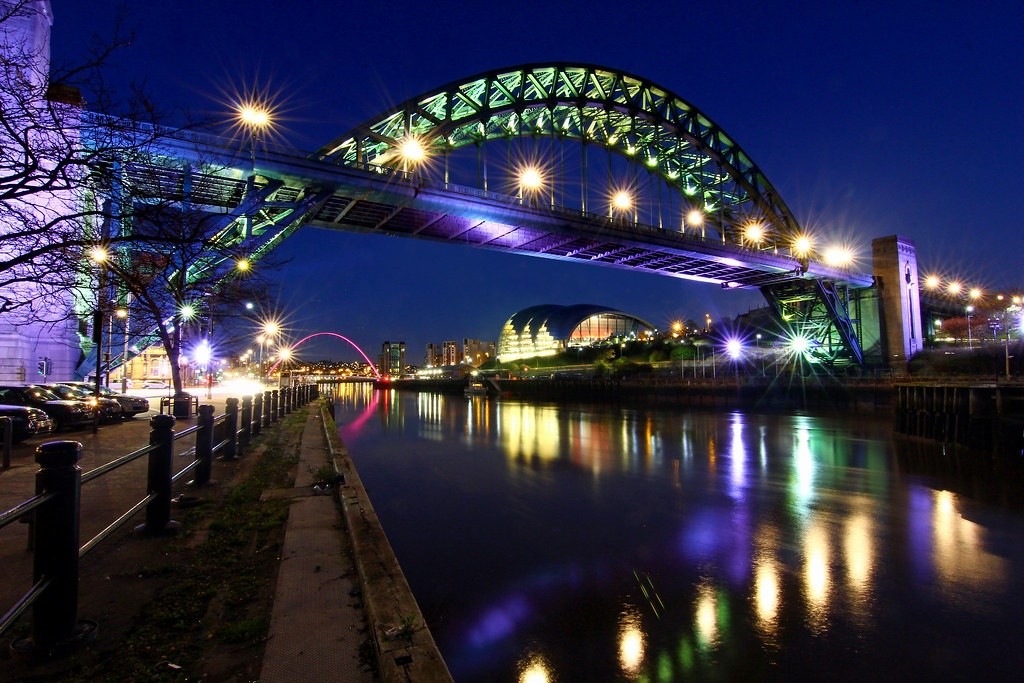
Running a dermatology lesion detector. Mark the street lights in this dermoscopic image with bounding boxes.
[237,319,294,381]
[1001,293,1021,375]
[86,247,111,385]
[966,305,975,349]
[179,302,195,388]
[116,310,129,393]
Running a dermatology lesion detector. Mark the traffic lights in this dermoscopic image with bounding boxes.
[49,359,55,375]
[38,360,46,376]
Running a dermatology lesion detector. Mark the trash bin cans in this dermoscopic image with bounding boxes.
[174,392,193,420]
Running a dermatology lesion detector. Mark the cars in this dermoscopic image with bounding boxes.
[44,384,122,422]
[1,383,100,429]
[143,379,174,389]
[66,381,149,416]
[0,403,53,438]
[116,378,133,389]
